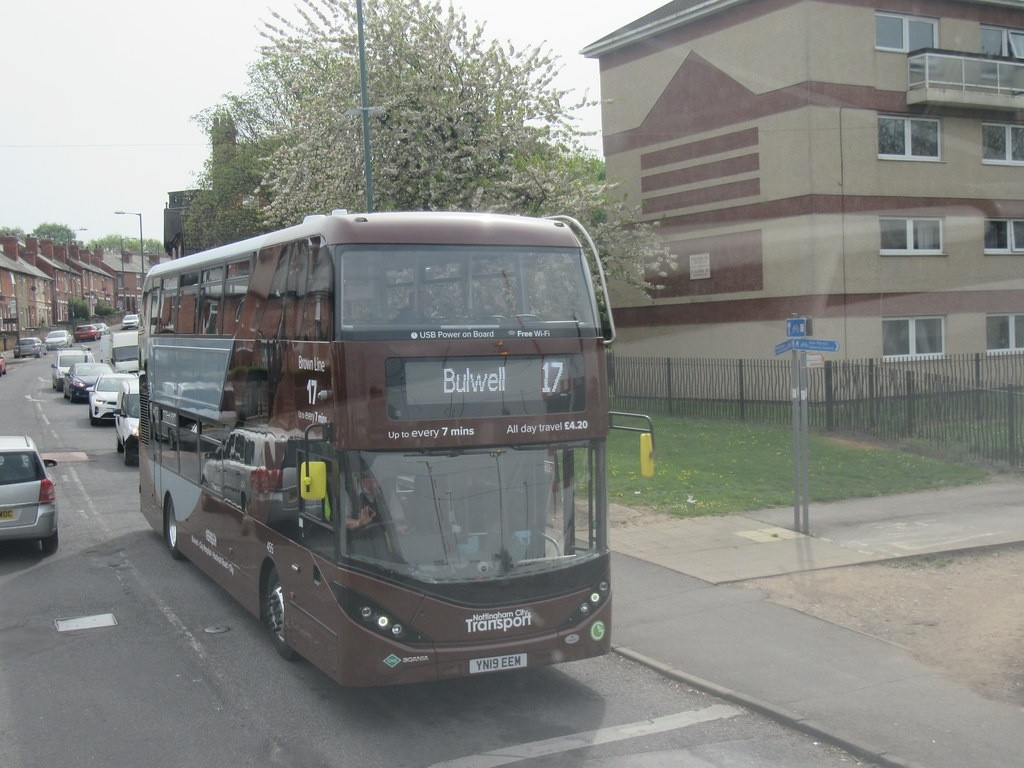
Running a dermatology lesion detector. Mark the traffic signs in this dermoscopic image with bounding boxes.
[774,338,792,356]
[792,339,840,351]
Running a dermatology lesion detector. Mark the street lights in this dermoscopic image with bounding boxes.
[114,210,145,284]
[120,238,137,315]
[66,227,87,327]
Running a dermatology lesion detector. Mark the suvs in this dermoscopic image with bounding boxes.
[0,435,60,554]
[199,429,325,524]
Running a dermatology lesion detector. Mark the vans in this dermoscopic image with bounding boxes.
[150,381,238,451]
[112,379,140,468]
[51,350,96,392]
[101,331,138,373]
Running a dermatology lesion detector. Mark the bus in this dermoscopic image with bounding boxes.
[136,210,655,688]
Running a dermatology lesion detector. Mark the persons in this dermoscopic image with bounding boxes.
[337,465,413,535]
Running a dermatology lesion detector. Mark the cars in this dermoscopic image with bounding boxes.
[75,325,102,343]
[63,363,114,404]
[12,337,47,359]
[91,323,111,340]
[86,374,140,426]
[44,330,74,351]
[121,315,140,331]
[0,354,7,377]
